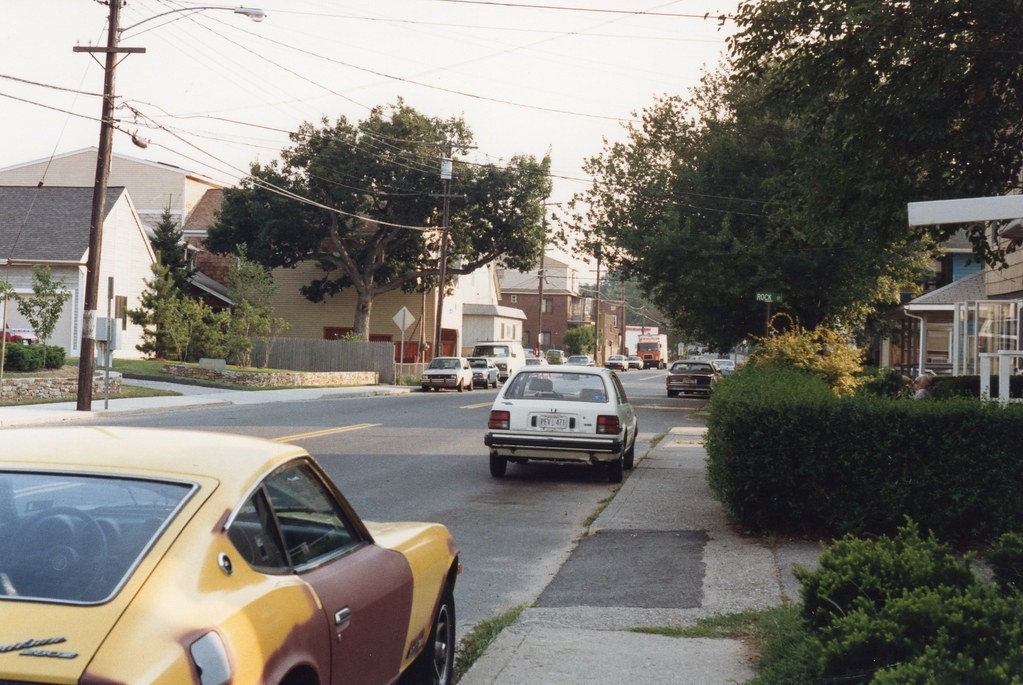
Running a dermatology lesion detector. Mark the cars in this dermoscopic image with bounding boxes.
[419,356,473,392]
[484,366,638,482]
[523,349,545,358]
[565,356,595,367]
[526,358,548,364]
[544,350,564,365]
[666,361,722,398]
[605,355,629,372]
[466,357,500,389]
[628,356,644,370]
[714,359,735,375]
[0,423,462,685]
[689,356,708,361]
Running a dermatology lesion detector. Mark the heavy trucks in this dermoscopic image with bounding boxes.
[636,334,668,370]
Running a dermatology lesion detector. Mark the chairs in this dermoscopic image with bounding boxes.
[580,389,605,402]
[529,378,563,398]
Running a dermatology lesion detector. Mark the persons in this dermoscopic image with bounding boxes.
[912,376,928,400]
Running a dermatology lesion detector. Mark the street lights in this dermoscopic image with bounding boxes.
[78,5,266,410]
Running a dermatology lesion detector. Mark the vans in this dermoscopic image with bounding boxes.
[472,342,525,383]
